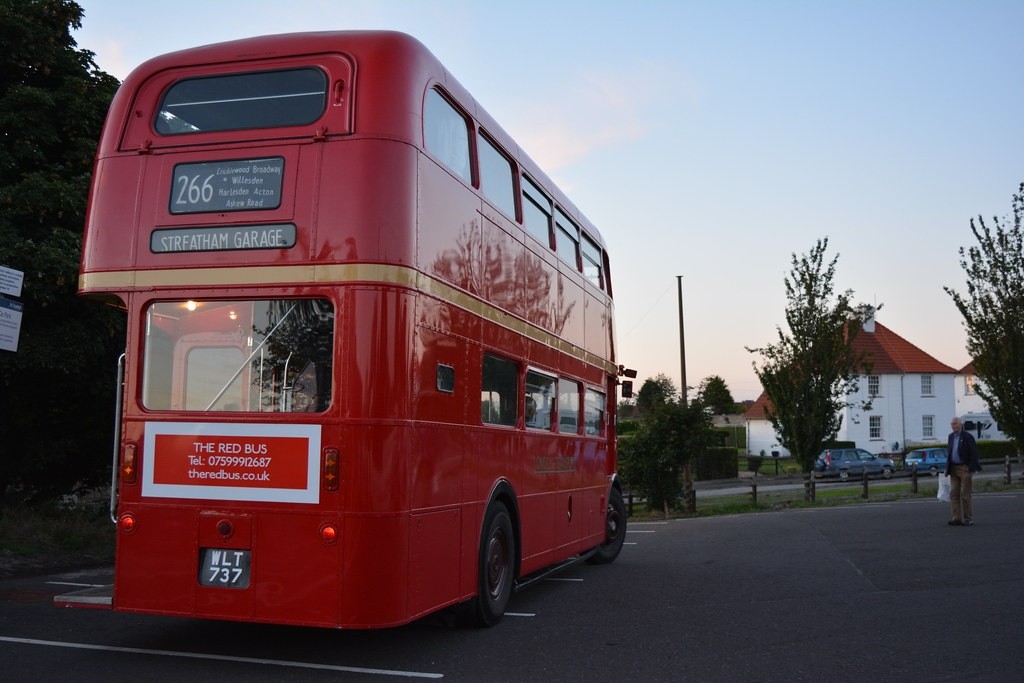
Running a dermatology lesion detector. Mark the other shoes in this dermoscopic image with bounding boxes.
[948,519,963,526]
[963,520,974,526]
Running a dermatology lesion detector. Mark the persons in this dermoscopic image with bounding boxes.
[523,396,538,427]
[943,417,982,526]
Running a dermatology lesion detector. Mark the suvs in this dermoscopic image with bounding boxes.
[814,449,896,482]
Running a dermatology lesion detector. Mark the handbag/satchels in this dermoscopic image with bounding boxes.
[936,472,951,502]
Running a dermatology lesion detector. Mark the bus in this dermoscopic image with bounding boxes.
[53,29,639,632]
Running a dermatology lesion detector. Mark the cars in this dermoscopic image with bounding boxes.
[905,448,949,476]
